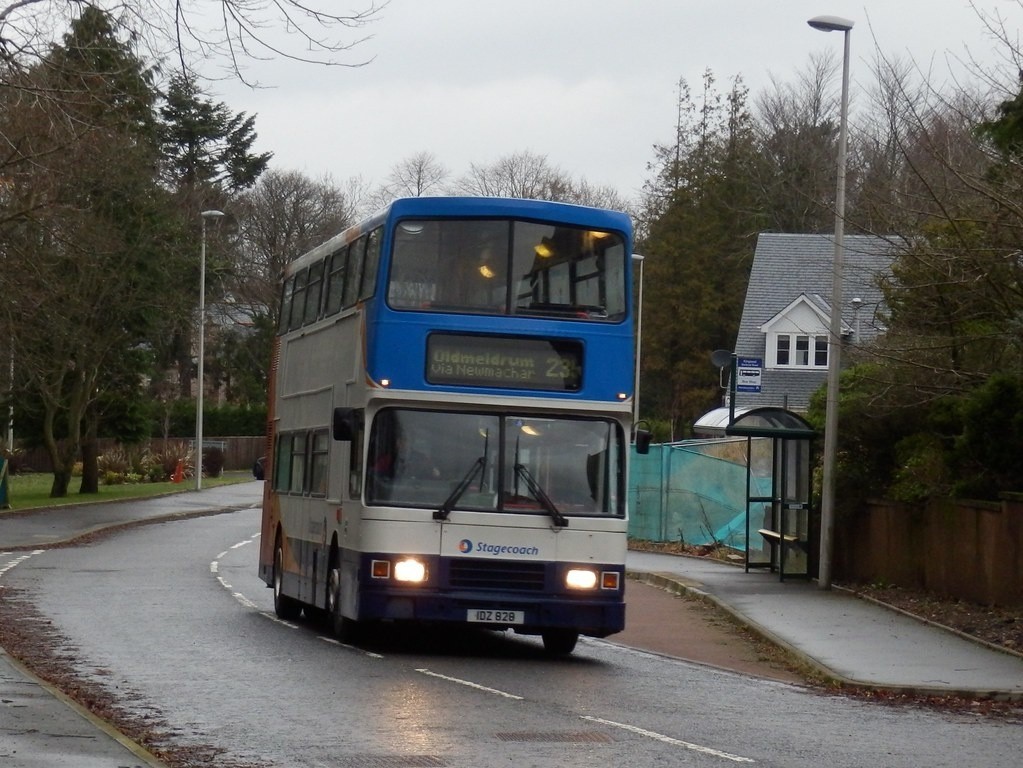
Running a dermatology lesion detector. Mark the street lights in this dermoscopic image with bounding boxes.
[195,210,225,491]
[7,300,17,451]
[808,15,854,592]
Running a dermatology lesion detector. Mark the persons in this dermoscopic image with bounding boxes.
[421,260,486,309]
[374,426,448,502]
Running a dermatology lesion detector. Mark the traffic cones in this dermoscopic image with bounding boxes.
[173,463,182,483]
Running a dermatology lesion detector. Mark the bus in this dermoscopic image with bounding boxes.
[259,196,653,653]
